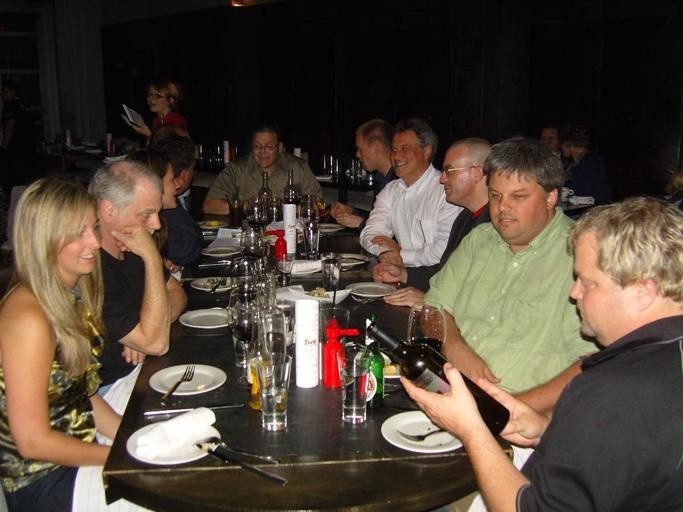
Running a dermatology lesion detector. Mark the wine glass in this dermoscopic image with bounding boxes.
[231,199,276,386]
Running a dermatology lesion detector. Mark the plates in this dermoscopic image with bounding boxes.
[200,246,243,258]
[126,420,222,466]
[178,309,228,329]
[291,259,321,276]
[343,281,397,298]
[317,223,345,233]
[341,253,368,266]
[197,220,229,231]
[190,276,238,292]
[284,288,352,304]
[149,364,228,396]
[381,411,463,453]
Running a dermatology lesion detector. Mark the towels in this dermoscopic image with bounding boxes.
[292,259,322,274]
[104,154,128,163]
[276,284,304,300]
[134,405,218,458]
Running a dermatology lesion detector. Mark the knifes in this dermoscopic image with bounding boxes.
[196,444,288,488]
[142,402,244,416]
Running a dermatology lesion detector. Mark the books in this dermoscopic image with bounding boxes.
[122,105,144,129]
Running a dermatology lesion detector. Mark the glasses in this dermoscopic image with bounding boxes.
[445,167,465,178]
[252,143,278,151]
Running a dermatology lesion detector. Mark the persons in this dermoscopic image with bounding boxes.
[204,126,324,215]
[420,136,602,413]
[399,194,683,512]
[0,177,123,512]
[132,79,202,275]
[540,127,603,204]
[0,79,35,251]
[87,159,189,386]
[329,119,492,310]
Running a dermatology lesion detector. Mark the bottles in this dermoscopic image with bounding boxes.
[364,312,511,436]
[257,171,272,199]
[282,169,301,217]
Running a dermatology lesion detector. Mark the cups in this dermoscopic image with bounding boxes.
[303,227,320,255]
[321,253,342,291]
[276,253,295,285]
[336,347,371,425]
[407,303,446,354]
[257,353,292,432]
[321,308,349,355]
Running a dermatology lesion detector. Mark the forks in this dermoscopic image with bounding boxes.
[160,364,195,405]
[395,429,448,443]
